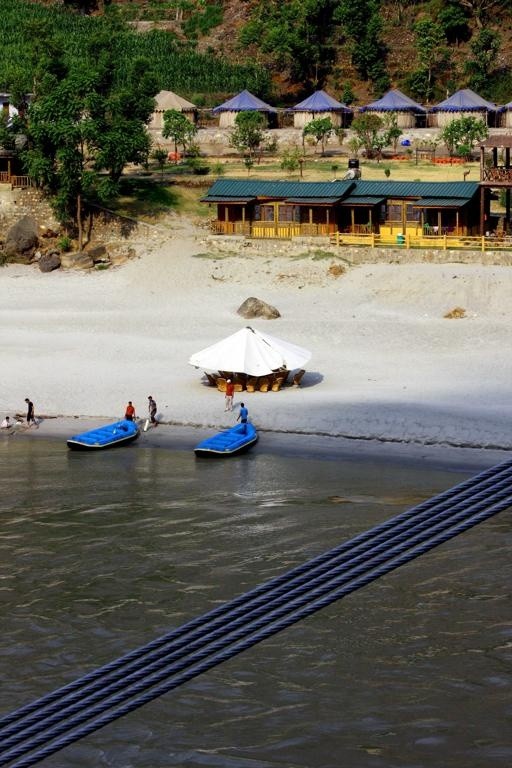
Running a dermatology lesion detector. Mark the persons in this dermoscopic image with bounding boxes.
[236,402,249,423]
[13,414,24,426]
[1,416,13,429]
[224,378,235,412]
[148,396,159,426]
[124,400,137,422]
[24,397,39,431]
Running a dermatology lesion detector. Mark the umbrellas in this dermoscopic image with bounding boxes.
[189,325,312,376]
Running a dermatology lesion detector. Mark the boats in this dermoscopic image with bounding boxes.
[192,422,259,461]
[65,419,141,452]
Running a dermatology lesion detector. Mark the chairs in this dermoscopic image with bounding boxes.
[203,368,305,393]
